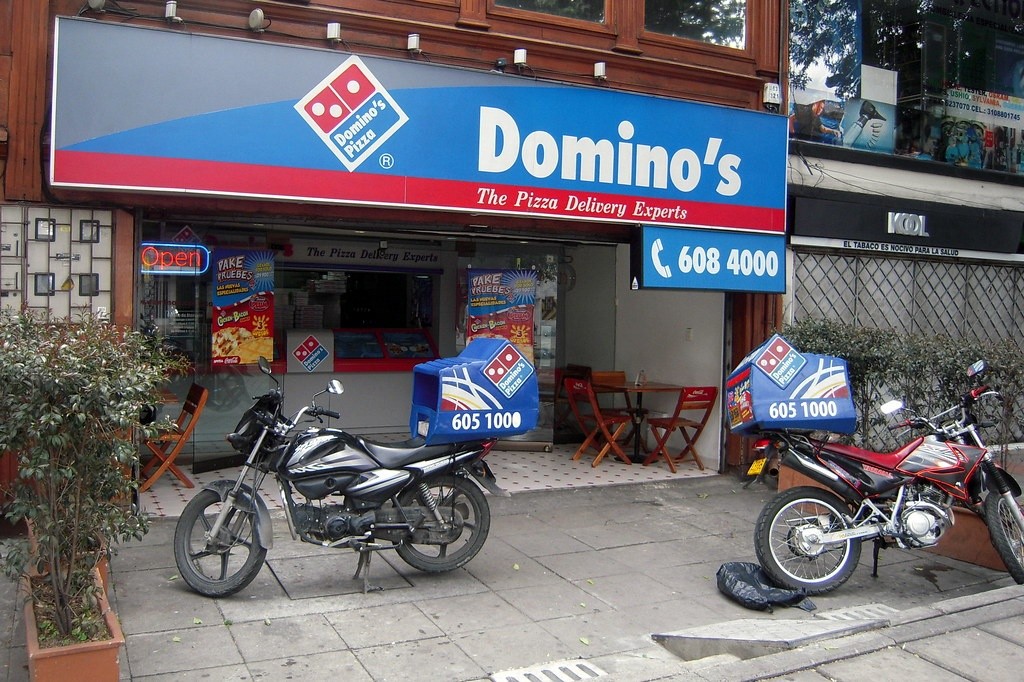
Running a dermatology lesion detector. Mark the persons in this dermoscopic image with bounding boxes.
[945,135,981,169]
[789,99,841,143]
[1008,138,1021,173]
[982,126,1010,171]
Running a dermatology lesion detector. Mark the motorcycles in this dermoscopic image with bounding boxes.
[736,359,1024,601]
[168,355,507,599]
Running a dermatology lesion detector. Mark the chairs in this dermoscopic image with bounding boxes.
[138,384,209,494]
[552,363,718,473]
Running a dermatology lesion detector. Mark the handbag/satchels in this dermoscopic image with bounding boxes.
[716,563,817,614]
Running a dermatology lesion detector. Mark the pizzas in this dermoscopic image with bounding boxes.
[212,327,253,357]
[226,337,273,363]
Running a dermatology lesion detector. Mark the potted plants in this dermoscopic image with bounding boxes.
[768,315,1024,571]
[0,300,200,682]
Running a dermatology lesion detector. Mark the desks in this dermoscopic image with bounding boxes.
[591,380,684,464]
[141,387,180,423]
